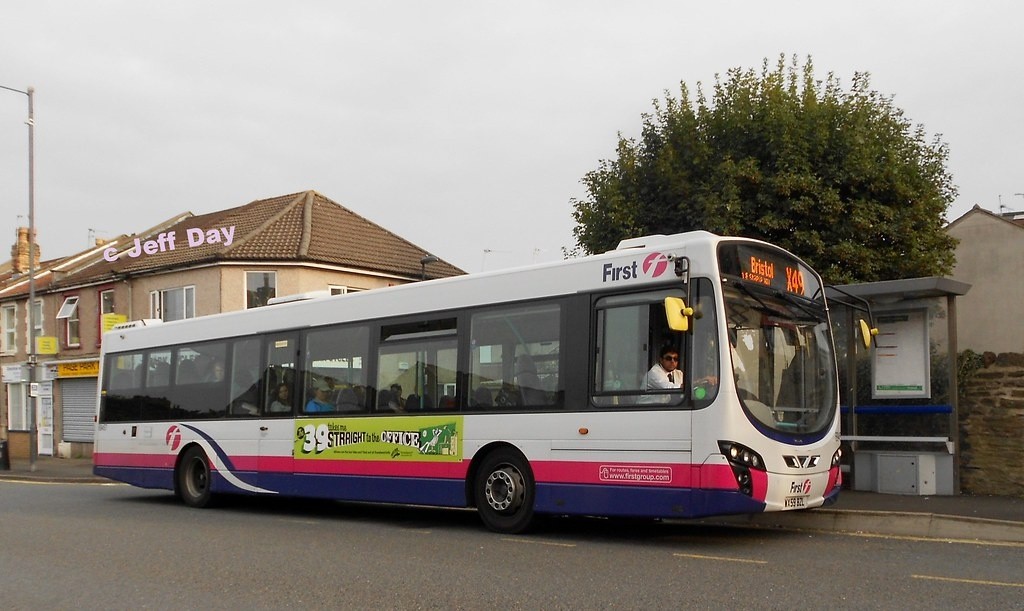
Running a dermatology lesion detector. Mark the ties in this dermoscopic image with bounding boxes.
[668,374,672,382]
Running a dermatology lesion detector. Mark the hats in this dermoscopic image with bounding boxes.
[312,379,332,392]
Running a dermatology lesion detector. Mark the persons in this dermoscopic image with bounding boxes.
[189,356,407,414]
[637,347,717,404]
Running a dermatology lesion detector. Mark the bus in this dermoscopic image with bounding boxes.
[88,230,880,538]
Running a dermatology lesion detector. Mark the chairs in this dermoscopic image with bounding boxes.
[337,388,361,412]
[476,386,492,406]
[515,354,547,406]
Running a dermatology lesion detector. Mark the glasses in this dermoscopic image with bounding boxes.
[663,356,680,362]
